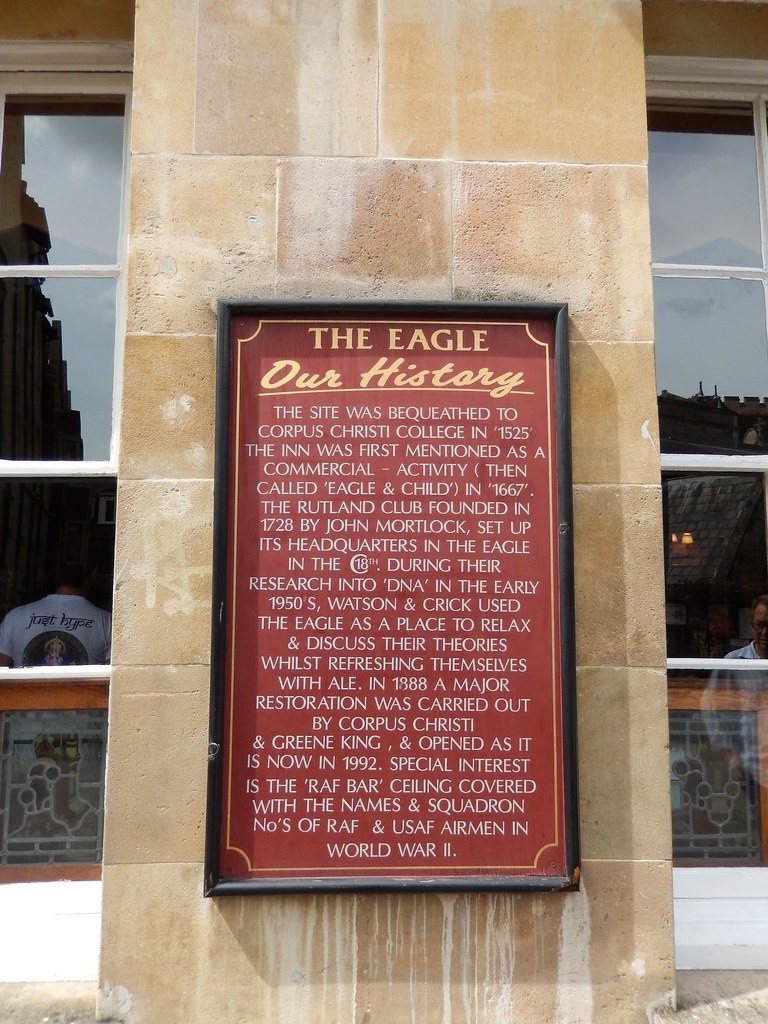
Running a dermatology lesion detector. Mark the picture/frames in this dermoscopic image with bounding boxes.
[203,298,580,902]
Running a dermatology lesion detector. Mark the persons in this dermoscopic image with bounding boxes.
[0,550,111,859]
[700,597,768,839]
